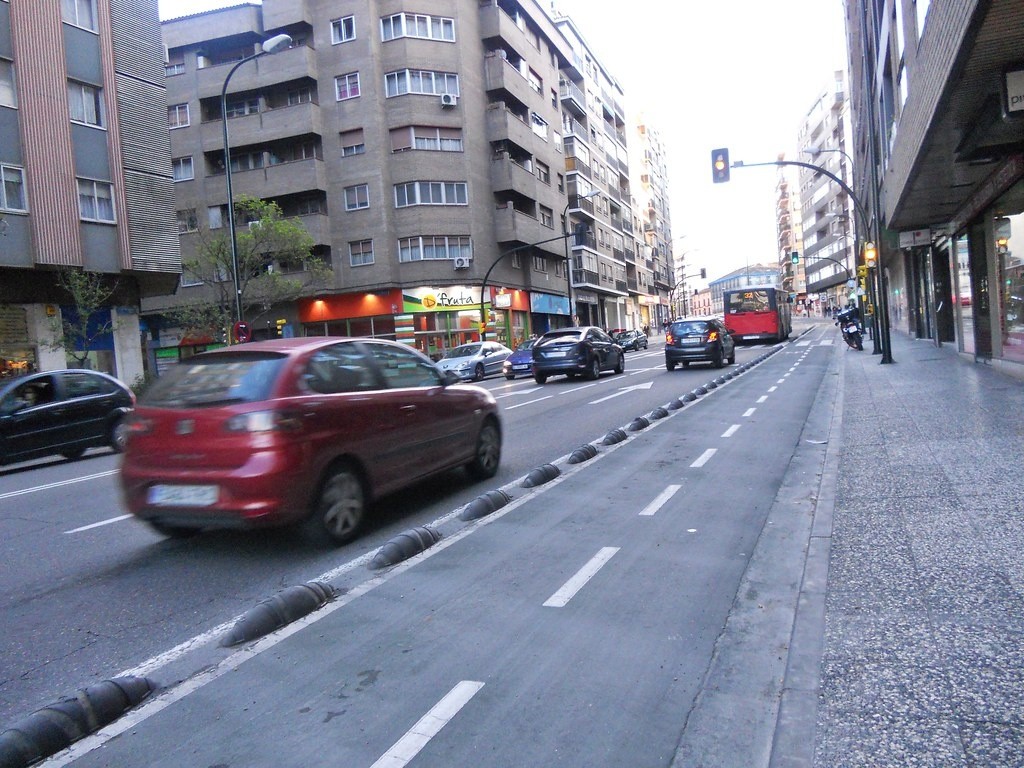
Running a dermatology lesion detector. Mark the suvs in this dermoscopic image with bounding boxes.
[530,327,625,384]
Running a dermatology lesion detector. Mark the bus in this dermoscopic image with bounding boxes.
[723,287,793,345]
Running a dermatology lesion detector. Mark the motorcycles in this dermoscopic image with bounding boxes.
[833,309,863,352]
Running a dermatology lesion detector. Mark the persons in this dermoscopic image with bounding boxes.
[663,319,672,333]
[822,301,855,318]
[644,323,649,338]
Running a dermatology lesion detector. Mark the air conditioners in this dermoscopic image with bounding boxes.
[441,93,456,106]
[454,257,469,268]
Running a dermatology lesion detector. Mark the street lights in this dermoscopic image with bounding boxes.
[221,34,292,322]
[803,147,867,334]
[261,305,272,338]
[675,289,699,321]
[563,190,602,326]
[825,213,866,334]
[666,235,685,298]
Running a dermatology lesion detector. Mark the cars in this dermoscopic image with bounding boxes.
[615,330,649,353]
[1006,296,1022,306]
[0,369,137,466]
[429,341,514,382]
[664,317,735,371]
[952,290,972,305]
[122,337,503,543]
[502,338,554,380]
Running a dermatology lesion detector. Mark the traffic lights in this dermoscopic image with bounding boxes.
[792,252,799,264]
[997,237,1008,254]
[276,319,287,336]
[856,265,867,292]
[867,304,873,314]
[712,147,730,184]
[673,304,676,310]
[864,241,877,269]
